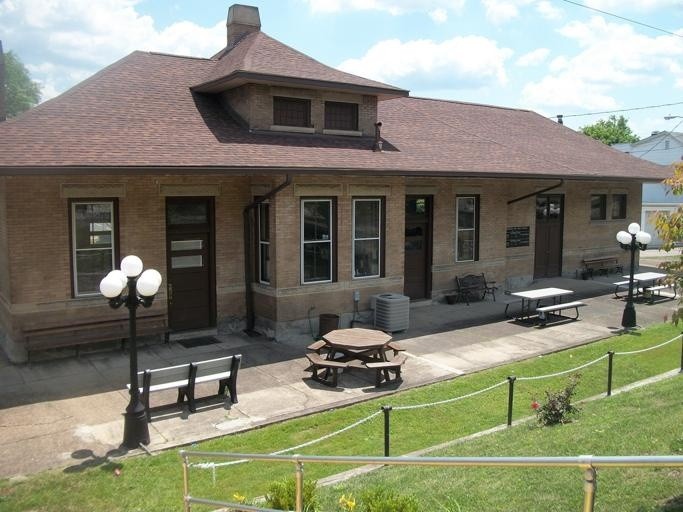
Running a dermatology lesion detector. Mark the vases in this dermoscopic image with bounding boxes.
[445,294,457,304]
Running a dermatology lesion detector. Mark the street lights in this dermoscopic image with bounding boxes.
[98,256,162,447]
[614,222,651,328]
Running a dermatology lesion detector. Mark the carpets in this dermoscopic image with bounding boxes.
[176,335,224,349]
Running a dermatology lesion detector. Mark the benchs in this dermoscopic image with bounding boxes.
[503,299,588,325]
[126,354,242,423]
[23,305,173,362]
[305,340,409,387]
[612,280,679,305]
[584,254,625,280]
[455,272,498,306]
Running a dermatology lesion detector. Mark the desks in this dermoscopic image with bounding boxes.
[510,287,574,322]
[622,272,667,299]
[322,327,393,381]
